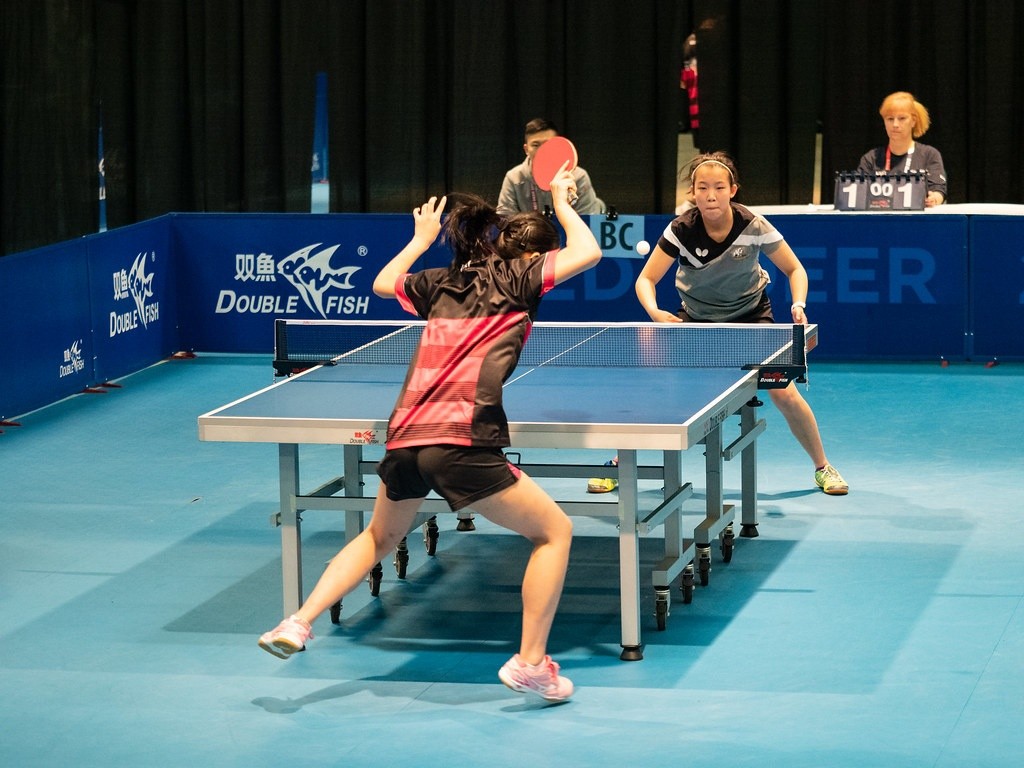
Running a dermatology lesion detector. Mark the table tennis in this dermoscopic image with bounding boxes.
[636,240,650,255]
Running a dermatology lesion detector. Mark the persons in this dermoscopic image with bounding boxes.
[854,91,947,208]
[495,119,608,216]
[587,153,850,496]
[258,157,603,704]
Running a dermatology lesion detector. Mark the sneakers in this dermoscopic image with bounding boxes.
[814,465,849,494]
[258,615,314,660]
[587,460,619,492]
[499,654,574,700]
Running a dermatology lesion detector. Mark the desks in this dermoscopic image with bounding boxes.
[743,202,1024,217]
[193,316,821,661]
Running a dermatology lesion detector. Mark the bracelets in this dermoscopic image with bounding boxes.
[791,302,806,314]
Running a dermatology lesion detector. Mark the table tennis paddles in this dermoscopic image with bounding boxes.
[532,136,579,206]
